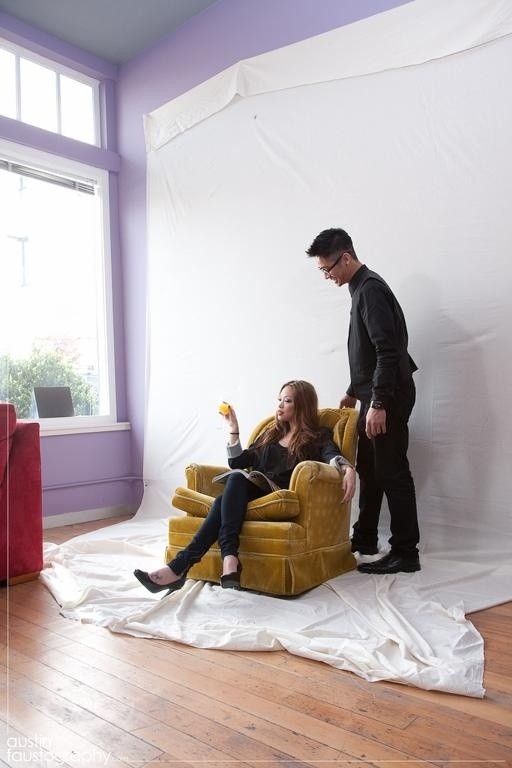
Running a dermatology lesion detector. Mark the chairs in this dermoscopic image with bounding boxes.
[166,409,360,596]
[1,402,42,591]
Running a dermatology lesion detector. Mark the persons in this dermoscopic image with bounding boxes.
[131,380,358,602]
[305,225,423,574]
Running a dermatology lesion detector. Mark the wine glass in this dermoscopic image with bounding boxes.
[218,399,233,432]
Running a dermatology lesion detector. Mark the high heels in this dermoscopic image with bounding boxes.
[219,558,243,589]
[134,567,187,600]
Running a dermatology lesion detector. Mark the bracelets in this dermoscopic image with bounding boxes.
[230,432,240,435]
[343,466,354,474]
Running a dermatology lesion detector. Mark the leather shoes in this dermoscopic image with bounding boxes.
[356,544,422,575]
[349,534,379,555]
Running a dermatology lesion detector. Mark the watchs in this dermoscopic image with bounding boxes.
[370,401,385,409]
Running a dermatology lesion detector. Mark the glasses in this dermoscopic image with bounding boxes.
[319,252,344,275]
